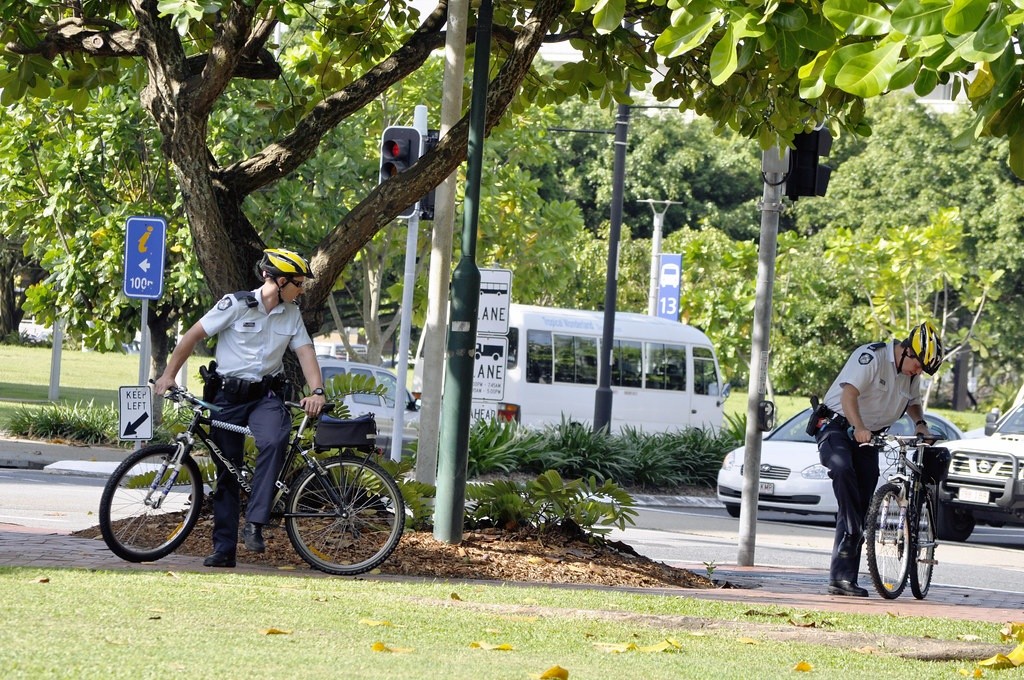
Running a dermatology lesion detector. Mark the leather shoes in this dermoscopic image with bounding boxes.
[827,578,868,597]
[836,529,863,557]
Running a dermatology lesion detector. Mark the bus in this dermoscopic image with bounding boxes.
[409,301,731,437]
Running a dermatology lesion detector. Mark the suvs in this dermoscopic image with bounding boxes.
[935,385,1024,540]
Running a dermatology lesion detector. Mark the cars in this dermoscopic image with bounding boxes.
[314,342,421,423]
[717,406,966,525]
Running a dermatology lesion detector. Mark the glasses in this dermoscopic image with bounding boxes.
[286,278,302,288]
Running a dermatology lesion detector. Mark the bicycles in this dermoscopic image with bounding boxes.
[99,377,404,575]
[847,425,939,600]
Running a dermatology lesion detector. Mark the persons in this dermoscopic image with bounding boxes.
[814,321,944,596]
[152,249,327,567]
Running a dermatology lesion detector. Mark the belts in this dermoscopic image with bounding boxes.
[828,411,850,426]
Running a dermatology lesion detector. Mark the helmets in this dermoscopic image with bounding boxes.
[907,320,943,376]
[259,248,310,279]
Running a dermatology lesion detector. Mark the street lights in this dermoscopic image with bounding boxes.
[637,196,682,374]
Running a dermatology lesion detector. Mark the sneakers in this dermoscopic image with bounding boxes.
[203,551,237,568]
[239,523,265,554]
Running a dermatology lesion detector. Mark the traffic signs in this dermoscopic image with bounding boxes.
[123,217,166,300]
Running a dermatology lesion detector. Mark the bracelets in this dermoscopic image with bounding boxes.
[916,419,927,427]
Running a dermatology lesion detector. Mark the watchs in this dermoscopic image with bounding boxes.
[312,387,324,395]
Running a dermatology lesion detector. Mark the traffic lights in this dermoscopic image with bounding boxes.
[783,127,834,198]
[378,125,423,223]
